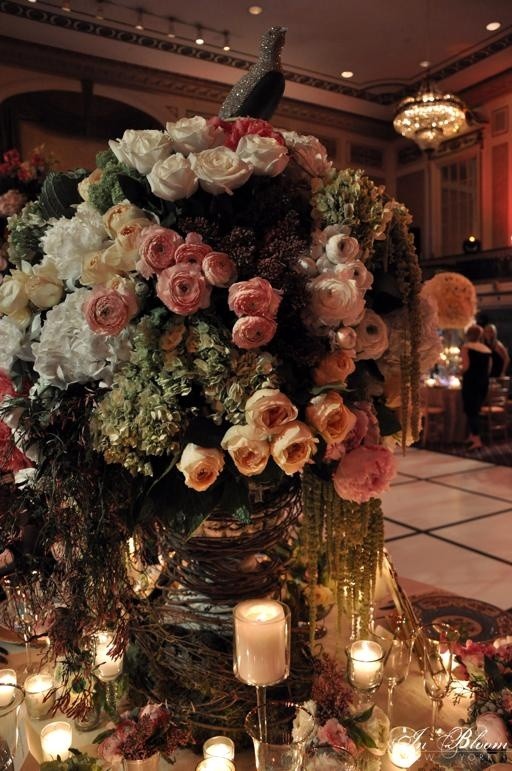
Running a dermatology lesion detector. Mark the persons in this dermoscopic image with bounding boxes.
[458,323,509,451]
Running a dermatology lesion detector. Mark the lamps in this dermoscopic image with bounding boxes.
[390,0,472,152]
[27,0,232,53]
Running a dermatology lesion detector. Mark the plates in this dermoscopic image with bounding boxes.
[0,595,57,645]
[390,595,512,649]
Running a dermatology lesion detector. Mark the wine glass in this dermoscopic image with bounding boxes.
[231,598,454,771]
[0,571,50,684]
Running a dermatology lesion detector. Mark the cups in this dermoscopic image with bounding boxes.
[40,721,73,763]
[0,684,26,771]
[0,668,17,706]
[22,674,56,719]
[194,736,236,771]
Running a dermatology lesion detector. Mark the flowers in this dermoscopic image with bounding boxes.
[0,116,512,771]
